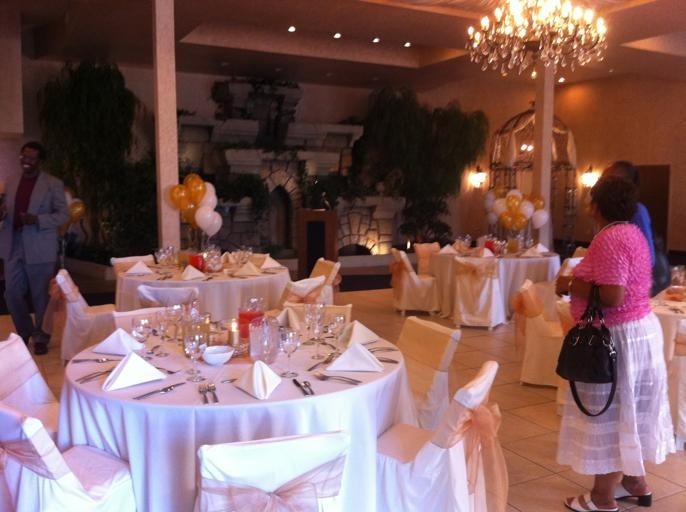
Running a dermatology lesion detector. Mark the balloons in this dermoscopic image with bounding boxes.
[169,172,224,238]
[65,185,86,222]
[483,189,548,234]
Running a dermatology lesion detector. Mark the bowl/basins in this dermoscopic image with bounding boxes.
[203,346,233,365]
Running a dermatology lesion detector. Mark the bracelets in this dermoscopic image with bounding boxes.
[568,275,577,288]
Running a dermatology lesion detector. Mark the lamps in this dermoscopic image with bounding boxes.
[472,164,485,189]
[581,165,598,188]
[465,0,608,78]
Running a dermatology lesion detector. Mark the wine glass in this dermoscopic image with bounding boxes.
[306,306,323,344]
[185,334,208,381]
[457,231,537,256]
[278,327,299,379]
[165,298,183,344]
[309,323,324,360]
[328,315,346,360]
[156,312,169,358]
[152,244,176,267]
[231,250,240,276]
[243,247,252,273]
[208,246,221,277]
[131,318,152,360]
[191,314,209,355]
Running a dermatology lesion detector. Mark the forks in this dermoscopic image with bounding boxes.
[316,372,360,385]
[206,384,218,404]
[307,357,333,372]
[197,385,208,405]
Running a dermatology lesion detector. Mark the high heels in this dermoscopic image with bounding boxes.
[563,493,619,512]
[614,482,652,506]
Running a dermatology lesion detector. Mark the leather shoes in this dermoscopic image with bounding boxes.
[34,342,48,354]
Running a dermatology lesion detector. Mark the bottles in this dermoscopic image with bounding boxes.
[238,296,265,354]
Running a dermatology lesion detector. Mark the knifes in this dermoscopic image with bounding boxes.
[75,370,110,383]
[291,378,308,397]
[132,382,184,400]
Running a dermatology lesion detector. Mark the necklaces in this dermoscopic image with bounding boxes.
[591,221,636,241]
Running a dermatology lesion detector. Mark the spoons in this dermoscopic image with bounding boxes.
[146,344,159,354]
[73,356,108,365]
[301,380,313,396]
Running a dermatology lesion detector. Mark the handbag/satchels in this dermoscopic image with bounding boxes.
[652,233,670,298]
[555,285,616,416]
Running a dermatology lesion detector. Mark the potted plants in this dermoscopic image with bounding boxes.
[376,86,490,248]
[36,56,140,264]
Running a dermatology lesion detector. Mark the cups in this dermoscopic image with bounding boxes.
[220,320,240,355]
[250,321,272,365]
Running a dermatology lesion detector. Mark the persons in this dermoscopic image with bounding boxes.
[554,177,681,512]
[0,141,69,354]
[596,161,658,271]
